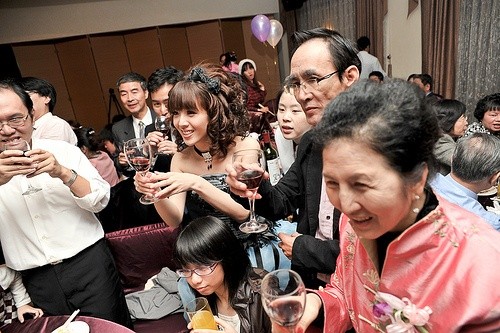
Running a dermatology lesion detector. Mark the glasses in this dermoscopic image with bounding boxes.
[176,262,218,278]
[0,111,30,132]
[284,71,339,95]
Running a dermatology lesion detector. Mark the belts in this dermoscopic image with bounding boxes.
[49,240,101,266]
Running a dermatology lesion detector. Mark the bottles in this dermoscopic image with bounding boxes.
[261,129,281,186]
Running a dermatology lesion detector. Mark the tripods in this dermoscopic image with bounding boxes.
[107,88,126,123]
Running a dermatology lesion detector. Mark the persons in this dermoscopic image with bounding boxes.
[431,132,500,233]
[368,71,384,84]
[0,80,128,324]
[269,78,500,333]
[431,98,468,175]
[220,51,273,139]
[133,63,297,320]
[224,28,362,333]
[356,36,387,79]
[270,85,312,174]
[465,93,500,211]
[407,74,443,98]
[68,66,188,234]
[175,216,285,333]
[0,264,43,328]
[18,77,78,146]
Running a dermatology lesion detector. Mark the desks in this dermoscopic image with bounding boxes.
[0,315,136,333]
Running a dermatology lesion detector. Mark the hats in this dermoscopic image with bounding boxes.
[238,59,256,77]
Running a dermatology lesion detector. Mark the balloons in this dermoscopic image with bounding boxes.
[266,20,284,46]
[251,14,271,42]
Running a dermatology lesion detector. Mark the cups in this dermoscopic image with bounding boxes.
[184,297,219,330]
[497,177,500,199]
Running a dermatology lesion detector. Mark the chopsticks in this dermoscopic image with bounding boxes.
[258,103,275,117]
[64,309,80,324]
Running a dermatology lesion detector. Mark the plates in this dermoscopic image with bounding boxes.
[477,186,497,196]
[50,321,90,333]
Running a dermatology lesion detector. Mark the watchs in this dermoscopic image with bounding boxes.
[63,170,77,187]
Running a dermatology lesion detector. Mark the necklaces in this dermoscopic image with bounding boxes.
[193,145,214,170]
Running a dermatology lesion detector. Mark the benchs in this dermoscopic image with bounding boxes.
[106,223,191,333]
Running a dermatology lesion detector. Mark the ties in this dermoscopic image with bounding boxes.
[138,121,146,156]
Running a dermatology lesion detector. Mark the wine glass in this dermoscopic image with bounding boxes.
[120,129,135,171]
[261,269,307,333]
[4,140,42,195]
[155,116,171,155]
[231,148,268,234]
[124,138,161,205]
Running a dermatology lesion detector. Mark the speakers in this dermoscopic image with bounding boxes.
[282,0,303,11]
